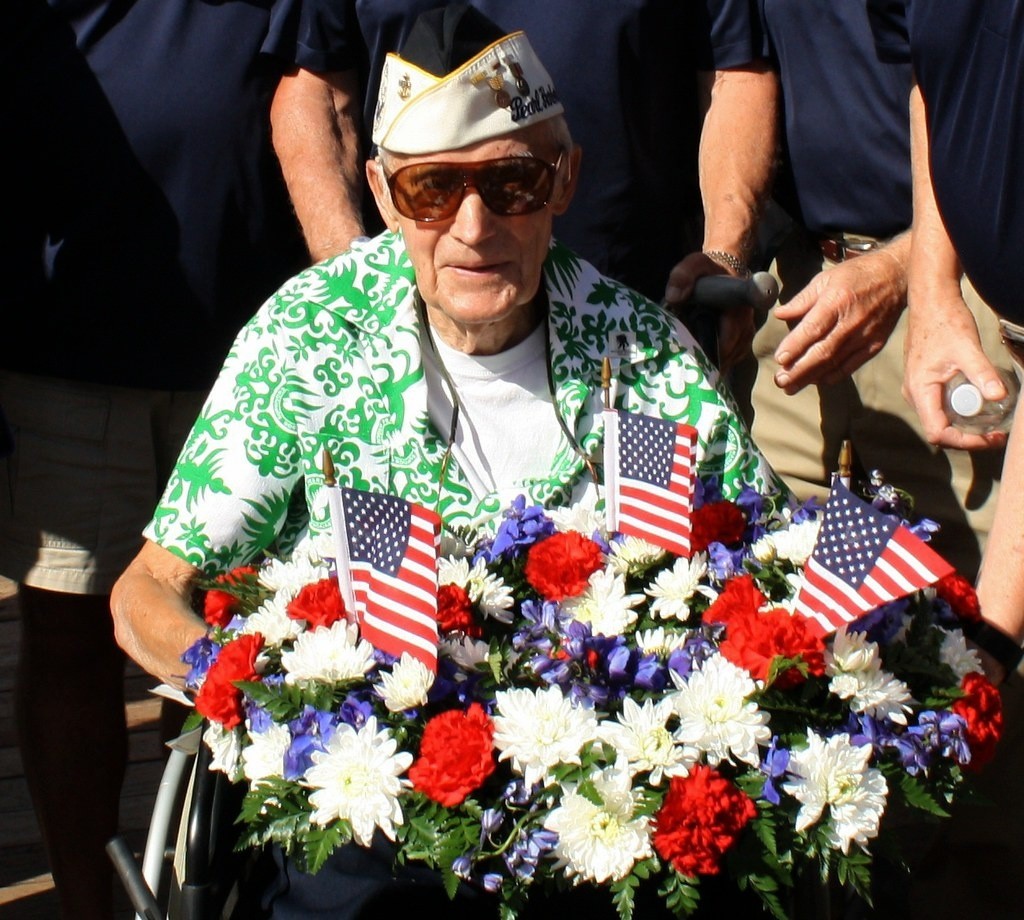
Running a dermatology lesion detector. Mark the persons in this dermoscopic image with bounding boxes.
[1,0,1024,920]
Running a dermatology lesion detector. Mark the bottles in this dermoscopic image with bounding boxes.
[944,366,1020,433]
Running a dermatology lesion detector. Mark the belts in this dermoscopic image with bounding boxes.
[790,223,864,264]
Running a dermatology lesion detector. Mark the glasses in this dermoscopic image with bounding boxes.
[381,149,564,221]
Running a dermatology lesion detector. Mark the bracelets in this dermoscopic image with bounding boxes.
[702,251,751,280]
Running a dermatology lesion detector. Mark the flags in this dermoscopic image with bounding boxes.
[789,470,957,641]
[603,407,699,558]
[331,487,443,681]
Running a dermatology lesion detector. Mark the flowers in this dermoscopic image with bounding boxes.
[190,464,1005,920]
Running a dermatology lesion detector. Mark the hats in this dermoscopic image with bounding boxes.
[371,5,563,154]
